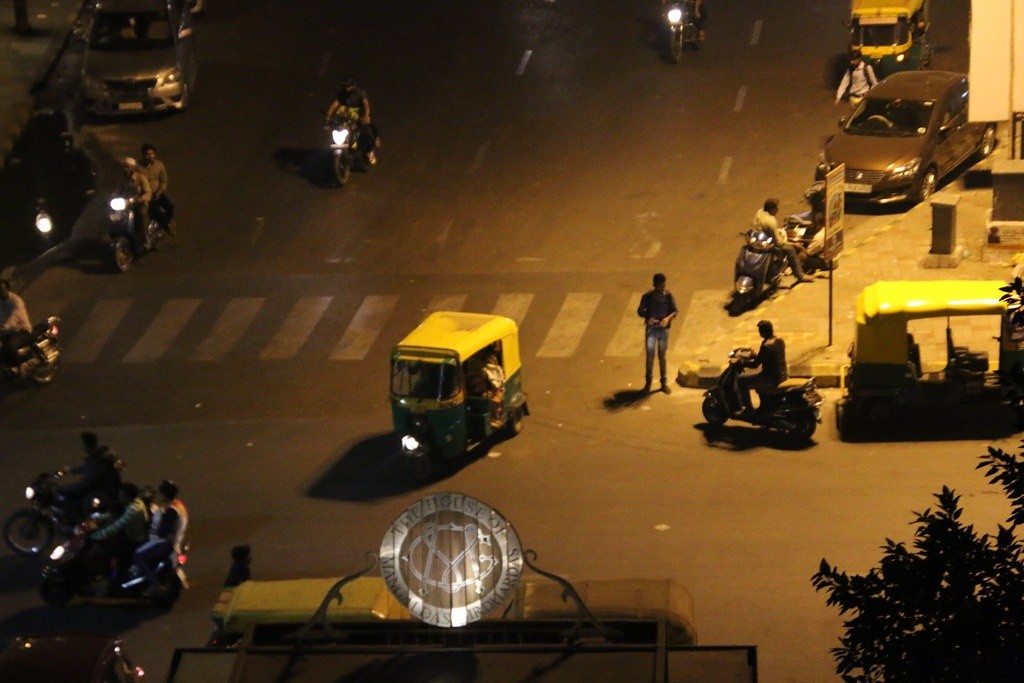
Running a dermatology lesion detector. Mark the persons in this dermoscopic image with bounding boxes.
[111,145,176,251]
[662,0,706,42]
[325,77,380,166]
[833,51,879,106]
[45,101,73,138]
[636,273,679,395]
[404,363,453,400]
[68,431,123,535]
[90,480,189,588]
[0,279,32,375]
[751,197,839,281]
[736,320,789,415]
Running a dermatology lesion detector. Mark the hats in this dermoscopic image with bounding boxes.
[123,157,135,165]
[159,480,179,499]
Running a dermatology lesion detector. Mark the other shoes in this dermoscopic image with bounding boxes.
[640,388,650,395]
[736,408,753,417]
[662,385,671,394]
[368,151,377,165]
[800,275,815,282]
[375,136,382,148]
[166,224,177,236]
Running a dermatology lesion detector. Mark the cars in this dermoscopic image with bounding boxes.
[80,0,198,121]
[814,70,998,213]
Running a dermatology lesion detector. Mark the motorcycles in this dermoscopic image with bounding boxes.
[6,465,191,610]
[319,121,378,185]
[844,0,932,76]
[388,309,529,481]
[834,280,1024,423]
[102,191,165,274]
[0,314,62,387]
[664,1,705,63]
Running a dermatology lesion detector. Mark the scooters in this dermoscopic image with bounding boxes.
[730,228,788,314]
[702,346,826,442]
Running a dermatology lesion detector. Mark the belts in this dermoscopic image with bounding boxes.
[850,94,864,98]
